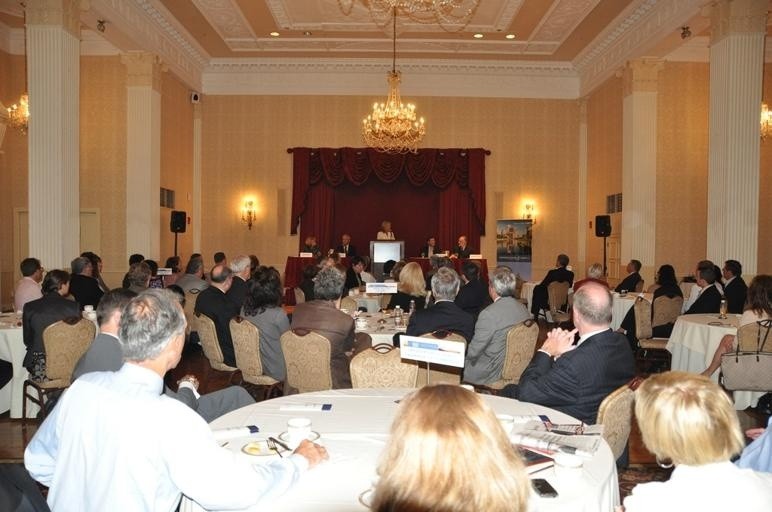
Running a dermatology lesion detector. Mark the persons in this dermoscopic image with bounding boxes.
[506,228,513,253]
[15,236,772,510]
[377,221,395,240]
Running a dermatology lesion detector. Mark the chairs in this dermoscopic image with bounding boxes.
[22,315,93,424]
[736,319,771,354]
[595,377,642,463]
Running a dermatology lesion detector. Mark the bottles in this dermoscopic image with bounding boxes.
[394,305,402,326]
[409,299,417,314]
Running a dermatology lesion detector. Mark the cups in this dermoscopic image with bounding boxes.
[718,300,727,319]
[356,319,367,329]
[496,414,514,435]
[16,304,23,319]
[287,417,312,448]
[444,251,450,257]
[84,305,94,311]
[401,309,409,327]
[621,290,628,297]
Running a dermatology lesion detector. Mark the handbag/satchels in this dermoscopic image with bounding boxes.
[722,353,771,391]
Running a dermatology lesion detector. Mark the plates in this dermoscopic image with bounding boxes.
[241,440,288,456]
[359,489,375,508]
[278,430,321,444]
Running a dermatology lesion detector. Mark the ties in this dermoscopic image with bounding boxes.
[345,246,347,256]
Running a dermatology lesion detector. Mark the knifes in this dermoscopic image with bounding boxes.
[268,436,292,451]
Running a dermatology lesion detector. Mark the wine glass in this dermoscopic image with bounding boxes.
[381,304,388,319]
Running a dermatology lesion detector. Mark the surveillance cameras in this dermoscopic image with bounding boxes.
[191,92,200,104]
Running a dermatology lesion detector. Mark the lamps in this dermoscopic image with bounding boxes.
[240,196,258,229]
[8,11,32,137]
[521,199,537,227]
[358,0,425,155]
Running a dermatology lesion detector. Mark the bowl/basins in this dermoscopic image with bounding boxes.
[551,452,583,468]
[82,311,96,320]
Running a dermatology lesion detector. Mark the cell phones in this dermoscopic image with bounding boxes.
[532,478,558,497]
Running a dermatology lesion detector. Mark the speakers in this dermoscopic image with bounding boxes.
[595,215,611,237]
[170,211,186,233]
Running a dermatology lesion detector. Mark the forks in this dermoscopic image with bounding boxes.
[267,439,283,458]
